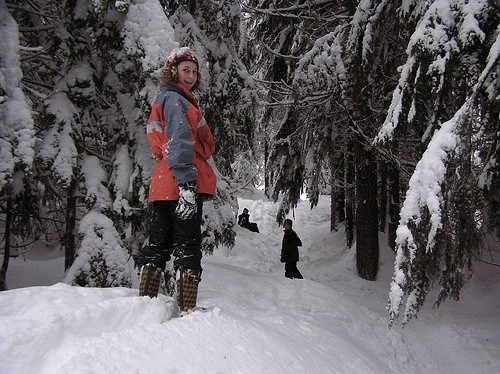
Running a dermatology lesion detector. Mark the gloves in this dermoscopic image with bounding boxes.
[175,181,198,220]
[280,256,287,262]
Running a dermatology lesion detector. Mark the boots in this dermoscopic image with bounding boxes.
[175,267,206,316]
[138,263,161,299]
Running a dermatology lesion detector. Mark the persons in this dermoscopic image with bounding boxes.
[139,48,217,317]
[238,208,260,233]
[280,219,304,279]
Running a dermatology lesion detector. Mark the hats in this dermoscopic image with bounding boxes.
[165,46,199,68]
